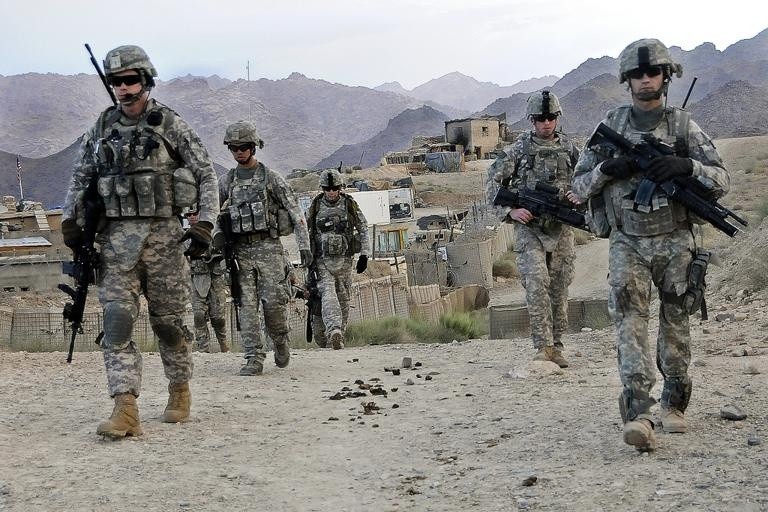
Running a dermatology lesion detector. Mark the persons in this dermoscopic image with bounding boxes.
[570,39,731,452]
[61,44,220,439]
[304,169,373,349]
[484,91,581,368]
[217,120,315,377]
[182,200,231,353]
[297,269,327,347]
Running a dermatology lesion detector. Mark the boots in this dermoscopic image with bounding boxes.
[97,390,144,439]
[218,339,230,353]
[239,356,264,376]
[330,328,344,351]
[273,341,290,368]
[658,396,688,434]
[550,345,568,368]
[163,379,193,424]
[197,343,211,354]
[622,417,659,452]
[534,342,553,361]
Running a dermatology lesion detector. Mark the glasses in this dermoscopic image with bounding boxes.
[186,212,199,217]
[228,144,251,152]
[623,65,661,80]
[109,74,141,88]
[532,113,558,122]
[322,185,341,192]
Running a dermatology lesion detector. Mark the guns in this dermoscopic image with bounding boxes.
[59,178,105,363]
[296,264,320,342]
[491,181,591,233]
[587,122,748,236]
[219,214,242,332]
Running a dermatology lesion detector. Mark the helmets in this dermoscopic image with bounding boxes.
[319,169,343,188]
[186,202,199,213]
[618,38,673,85]
[103,44,158,82]
[223,120,261,147]
[526,89,563,120]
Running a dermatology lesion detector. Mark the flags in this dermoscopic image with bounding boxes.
[15,157,23,184]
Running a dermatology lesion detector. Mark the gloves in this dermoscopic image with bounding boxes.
[644,153,693,183]
[355,254,368,274]
[177,218,214,259]
[298,248,314,268]
[600,158,634,180]
[61,218,85,250]
